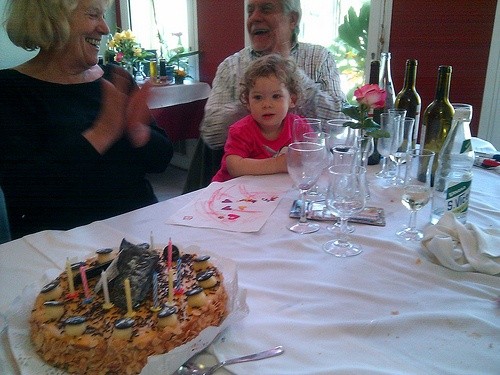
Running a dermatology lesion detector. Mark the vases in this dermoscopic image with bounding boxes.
[122,56,136,80]
[174,75,186,85]
[354,136,374,200]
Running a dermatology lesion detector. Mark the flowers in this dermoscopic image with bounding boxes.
[339,83,393,168]
[104,28,203,76]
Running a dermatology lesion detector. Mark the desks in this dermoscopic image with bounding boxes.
[134,76,213,177]
[0,136,500,375]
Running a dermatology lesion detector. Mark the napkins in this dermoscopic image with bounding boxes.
[419,210,500,276]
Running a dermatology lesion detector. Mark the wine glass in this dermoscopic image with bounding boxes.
[291,107,419,217]
[324,165,367,257]
[395,149,436,241]
[286,140,326,233]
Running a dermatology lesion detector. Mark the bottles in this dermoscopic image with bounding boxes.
[417,64,456,185]
[377,52,396,113]
[431,108,474,225]
[393,58,421,157]
[360,60,385,165]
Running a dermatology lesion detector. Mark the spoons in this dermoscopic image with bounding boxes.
[177,343,285,375]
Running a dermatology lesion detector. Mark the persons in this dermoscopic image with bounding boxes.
[0,0,172,241]
[201,0,352,151]
[212,54,319,182]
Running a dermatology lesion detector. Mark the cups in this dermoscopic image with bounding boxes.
[96,55,184,85]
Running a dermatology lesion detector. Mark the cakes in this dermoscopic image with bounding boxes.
[30,237,228,375]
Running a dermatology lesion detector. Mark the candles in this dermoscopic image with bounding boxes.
[168,266,174,304]
[152,271,158,309]
[124,276,133,314]
[65,256,76,294]
[167,237,173,273]
[78,265,91,300]
[148,230,154,252]
[101,270,111,305]
[176,257,182,290]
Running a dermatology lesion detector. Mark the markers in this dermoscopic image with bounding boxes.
[474,151,500,169]
